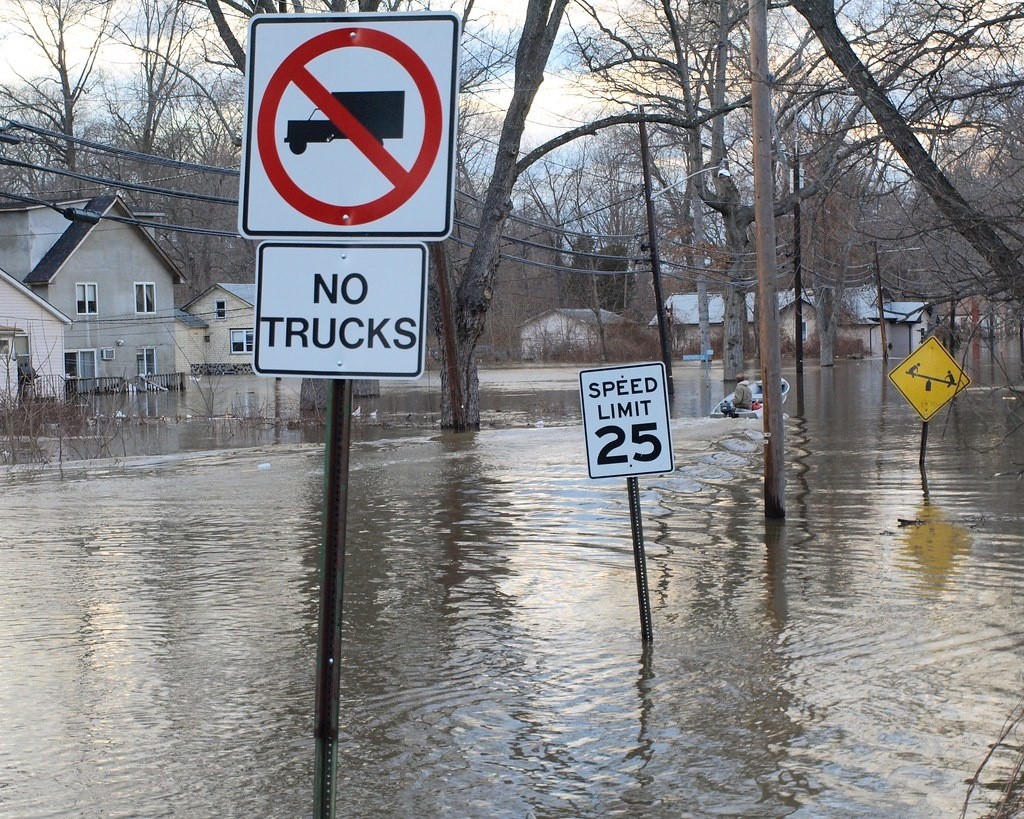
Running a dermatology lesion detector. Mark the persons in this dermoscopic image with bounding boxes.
[18,362,36,401]
[732,375,753,410]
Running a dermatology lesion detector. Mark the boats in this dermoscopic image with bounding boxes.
[708,376,791,420]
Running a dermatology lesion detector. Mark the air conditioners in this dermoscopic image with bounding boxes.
[101,348,116,360]
